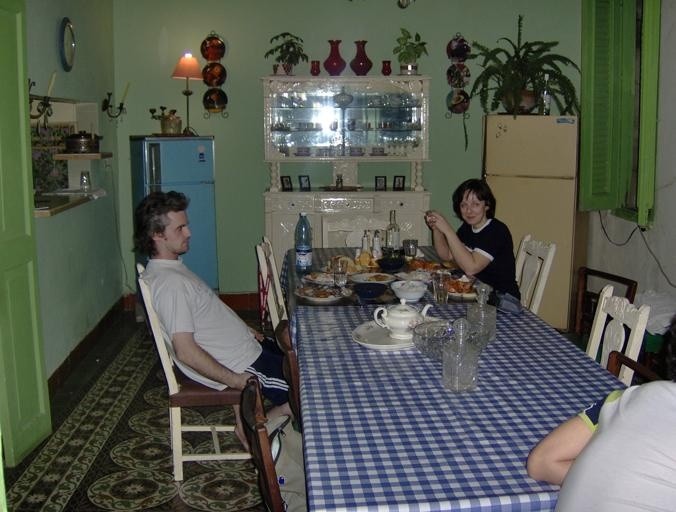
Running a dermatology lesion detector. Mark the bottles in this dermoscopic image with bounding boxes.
[336,174,343,189]
[293,211,313,275]
[440,316,481,391]
[464,284,498,339]
[541,74,552,117]
[385,209,401,253]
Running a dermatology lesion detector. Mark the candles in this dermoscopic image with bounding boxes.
[121,81,129,102]
[47,72,57,96]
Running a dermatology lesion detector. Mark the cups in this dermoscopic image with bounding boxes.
[78,170,91,188]
[327,114,412,129]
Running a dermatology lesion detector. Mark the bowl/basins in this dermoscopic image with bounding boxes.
[410,320,490,361]
[295,147,384,153]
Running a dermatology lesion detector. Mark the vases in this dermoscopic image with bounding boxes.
[332,86,353,106]
[349,40,372,75]
[323,39,346,76]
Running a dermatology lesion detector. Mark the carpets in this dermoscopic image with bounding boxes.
[6,308,284,511]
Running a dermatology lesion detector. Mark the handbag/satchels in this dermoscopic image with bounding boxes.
[265,415,308,512]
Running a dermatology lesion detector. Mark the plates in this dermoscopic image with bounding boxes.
[293,288,340,304]
[200,38,228,116]
[292,152,387,157]
[321,221,488,306]
[348,313,441,352]
[301,275,333,287]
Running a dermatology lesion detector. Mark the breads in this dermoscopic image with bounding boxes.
[359,251,370,266]
[411,259,440,271]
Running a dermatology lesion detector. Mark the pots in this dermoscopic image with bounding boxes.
[62,130,103,153]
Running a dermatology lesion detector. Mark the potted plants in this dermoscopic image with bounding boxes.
[264,31,308,75]
[460,14,581,153]
[392,27,429,75]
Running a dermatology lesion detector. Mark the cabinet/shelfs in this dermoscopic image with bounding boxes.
[256,76,433,279]
[34,150,112,216]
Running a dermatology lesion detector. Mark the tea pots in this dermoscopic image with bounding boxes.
[372,297,434,338]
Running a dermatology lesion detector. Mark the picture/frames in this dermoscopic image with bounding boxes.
[393,175,405,191]
[280,176,292,191]
[298,175,311,192]
[375,175,387,191]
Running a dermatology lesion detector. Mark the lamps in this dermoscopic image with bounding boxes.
[171,54,203,137]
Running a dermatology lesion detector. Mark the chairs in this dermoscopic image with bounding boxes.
[136,262,251,482]
[254,235,288,333]
[246,377,286,512]
[515,233,556,315]
[607,350,663,388]
[585,284,651,387]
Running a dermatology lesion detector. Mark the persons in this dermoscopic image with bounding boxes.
[424,179,521,303]
[130,191,293,451]
[526,313,676,512]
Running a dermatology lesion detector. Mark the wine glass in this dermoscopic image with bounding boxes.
[366,89,410,106]
[277,91,301,107]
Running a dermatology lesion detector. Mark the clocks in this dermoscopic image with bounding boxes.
[59,17,76,72]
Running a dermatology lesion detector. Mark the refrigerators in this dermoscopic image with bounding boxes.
[483,113,591,335]
[127,133,220,297]
